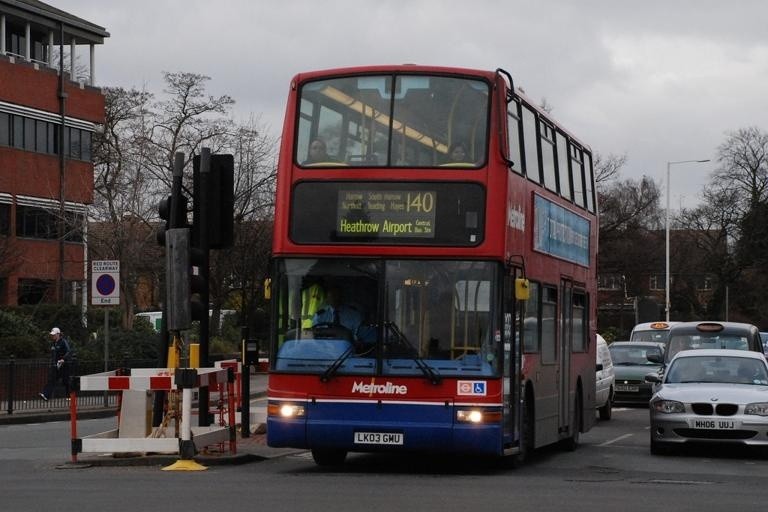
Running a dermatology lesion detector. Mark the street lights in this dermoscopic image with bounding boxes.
[664,159,712,322]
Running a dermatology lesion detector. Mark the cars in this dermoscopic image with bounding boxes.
[608,340,666,408]
[648,348,768,455]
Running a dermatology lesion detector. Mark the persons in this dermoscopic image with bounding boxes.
[312,286,365,345]
[38,327,72,401]
[305,137,342,162]
[439,142,474,164]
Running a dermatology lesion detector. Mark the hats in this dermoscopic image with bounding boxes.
[49,327,61,336]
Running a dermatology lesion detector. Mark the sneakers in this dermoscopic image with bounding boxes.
[39,393,47,400]
[66,398,71,400]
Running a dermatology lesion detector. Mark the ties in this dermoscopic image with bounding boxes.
[332,310,340,325]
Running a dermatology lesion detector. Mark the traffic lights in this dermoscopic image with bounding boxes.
[156,195,188,248]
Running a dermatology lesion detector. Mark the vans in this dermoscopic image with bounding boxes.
[596,331,616,421]
[628,320,768,372]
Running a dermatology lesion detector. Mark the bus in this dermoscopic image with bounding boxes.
[264,62,601,470]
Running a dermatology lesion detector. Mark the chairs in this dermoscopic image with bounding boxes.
[344,154,376,165]
